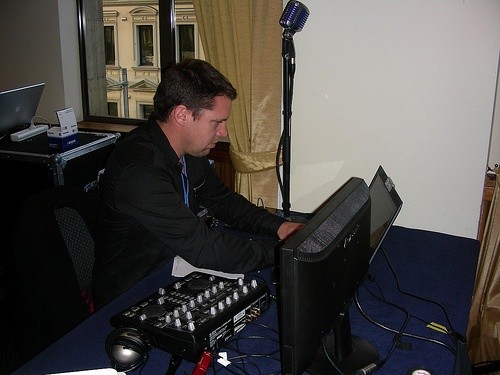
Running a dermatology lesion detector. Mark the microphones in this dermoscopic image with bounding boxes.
[279,0,310,32]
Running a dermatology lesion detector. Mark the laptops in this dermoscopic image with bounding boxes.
[368,165,403,264]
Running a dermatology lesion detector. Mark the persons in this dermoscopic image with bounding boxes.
[90,60,307,314]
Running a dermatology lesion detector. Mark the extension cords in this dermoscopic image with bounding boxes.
[10,124,48,142]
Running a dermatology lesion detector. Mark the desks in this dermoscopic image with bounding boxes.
[12,213,482,375]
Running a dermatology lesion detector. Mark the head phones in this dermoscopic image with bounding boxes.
[104,326,152,374]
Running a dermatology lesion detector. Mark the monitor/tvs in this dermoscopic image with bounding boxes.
[278,175,382,375]
[0,83,46,132]
